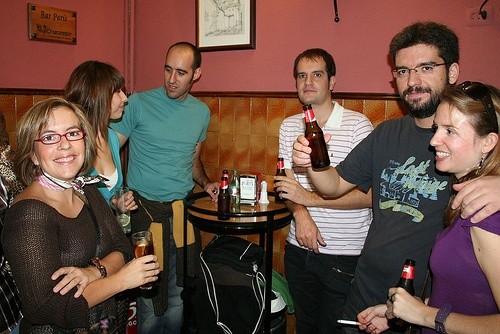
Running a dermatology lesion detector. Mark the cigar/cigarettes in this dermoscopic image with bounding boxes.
[336,319,362,326]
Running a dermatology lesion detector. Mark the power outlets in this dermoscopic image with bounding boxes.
[465,6,493,26]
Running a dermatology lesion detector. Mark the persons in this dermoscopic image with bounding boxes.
[0,96,160,334]
[61,60,139,245]
[273,47,374,334]
[292,20,500,334]
[107,41,221,317]
[358,81,500,334]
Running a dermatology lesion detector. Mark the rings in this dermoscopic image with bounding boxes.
[389,294,395,304]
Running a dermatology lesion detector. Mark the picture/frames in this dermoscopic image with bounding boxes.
[195,0,256,52]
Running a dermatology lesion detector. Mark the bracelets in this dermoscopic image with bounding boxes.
[86,258,107,279]
[434,303,453,334]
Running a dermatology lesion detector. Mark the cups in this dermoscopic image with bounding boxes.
[112,186,132,234]
[131,231,155,290]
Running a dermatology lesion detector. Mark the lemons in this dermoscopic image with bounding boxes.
[135,239,146,259]
[120,214,130,226]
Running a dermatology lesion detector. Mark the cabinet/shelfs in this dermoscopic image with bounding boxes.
[183,191,293,334]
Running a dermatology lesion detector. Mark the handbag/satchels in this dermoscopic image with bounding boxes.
[181,233,295,334]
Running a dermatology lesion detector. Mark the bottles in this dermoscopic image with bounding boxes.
[228,170,240,213]
[387,258,416,334]
[222,169,228,181]
[303,103,330,170]
[273,158,288,204]
[217,176,231,220]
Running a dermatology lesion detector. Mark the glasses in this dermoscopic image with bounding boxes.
[391,62,452,79]
[33,130,86,145]
[456,80,498,135]
